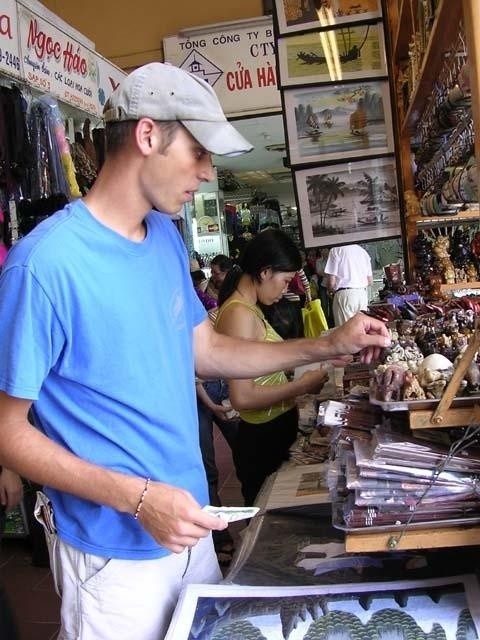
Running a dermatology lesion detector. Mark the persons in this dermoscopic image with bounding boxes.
[0,466,27,640]
[193,376,254,568]
[211,228,332,528]
[0,59,395,640]
[189,237,377,403]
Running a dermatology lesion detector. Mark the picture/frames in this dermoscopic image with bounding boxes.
[271,0,406,248]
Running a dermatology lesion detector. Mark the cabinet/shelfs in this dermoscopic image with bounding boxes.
[391,0,480,291]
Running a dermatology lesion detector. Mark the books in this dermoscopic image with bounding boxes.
[336,423,480,530]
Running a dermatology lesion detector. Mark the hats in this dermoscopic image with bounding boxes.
[102,62,256,157]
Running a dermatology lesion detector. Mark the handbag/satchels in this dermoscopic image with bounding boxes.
[291,272,305,295]
[301,299,329,338]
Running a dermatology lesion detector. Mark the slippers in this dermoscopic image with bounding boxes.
[216,547,235,567]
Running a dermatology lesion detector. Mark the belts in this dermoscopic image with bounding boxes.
[336,287,350,292]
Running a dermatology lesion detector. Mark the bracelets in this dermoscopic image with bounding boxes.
[134,478,150,521]
